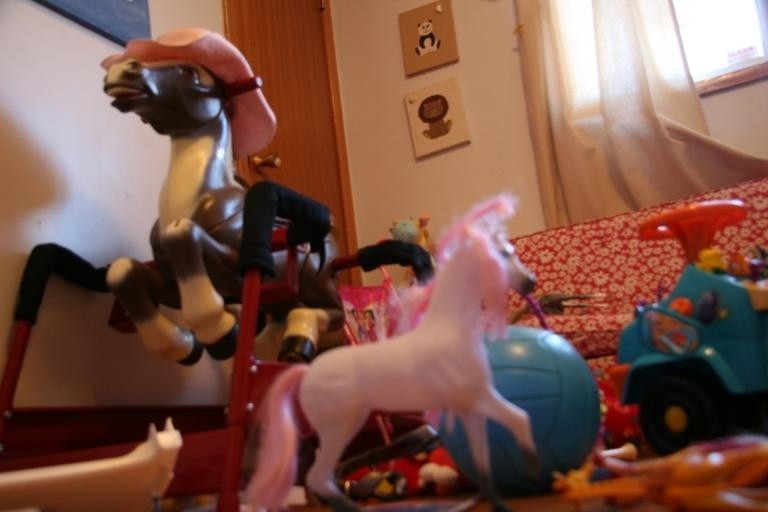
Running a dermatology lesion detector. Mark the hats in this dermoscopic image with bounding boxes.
[99,26,280,161]
